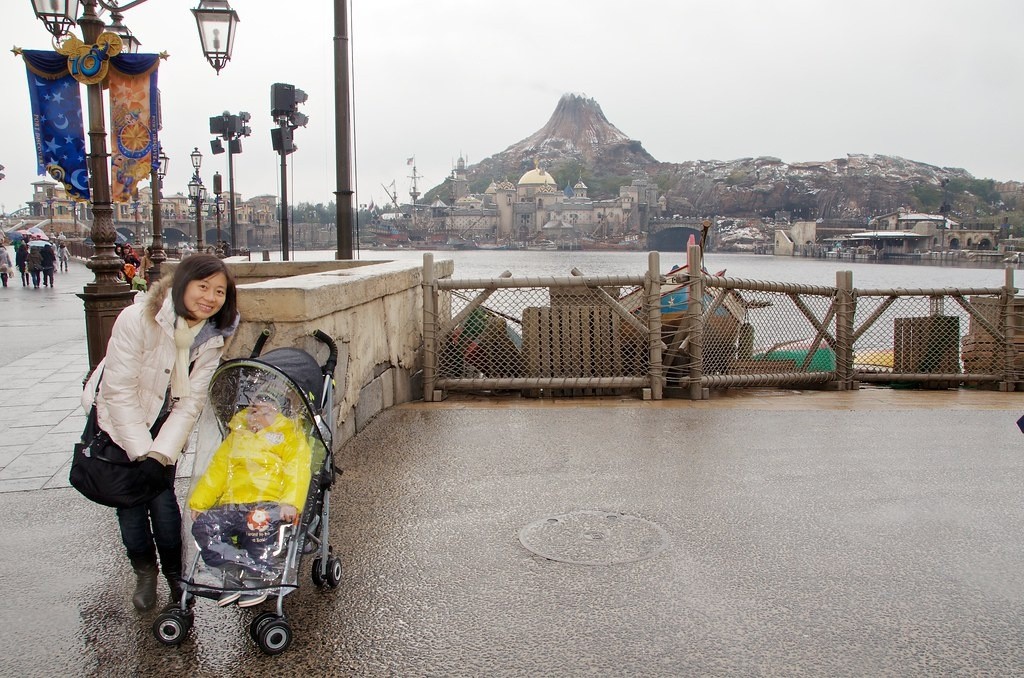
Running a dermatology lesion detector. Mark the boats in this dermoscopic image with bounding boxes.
[448,304,529,397]
[606,225,759,393]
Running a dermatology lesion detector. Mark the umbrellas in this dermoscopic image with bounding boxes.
[17,229,31,236]
[28,239,53,247]
[27,228,49,240]
[7,231,23,241]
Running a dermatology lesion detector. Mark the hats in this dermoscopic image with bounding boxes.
[250,379,287,413]
[45,242,52,246]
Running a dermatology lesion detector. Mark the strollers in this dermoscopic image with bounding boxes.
[150,326,346,657]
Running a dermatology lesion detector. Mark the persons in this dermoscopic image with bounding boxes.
[223,240,232,256]
[27,246,43,288]
[13,238,23,250]
[0,243,12,287]
[79,254,242,612]
[186,384,313,608]
[166,205,218,220]
[16,244,30,286]
[40,245,57,288]
[115,242,141,290]
[58,241,71,271]
[23,234,41,243]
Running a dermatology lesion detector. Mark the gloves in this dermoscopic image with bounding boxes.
[133,457,170,502]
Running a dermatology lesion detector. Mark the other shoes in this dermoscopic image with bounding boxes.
[217,576,241,606]
[242,580,272,607]
[51,284,53,287]
[43,282,47,286]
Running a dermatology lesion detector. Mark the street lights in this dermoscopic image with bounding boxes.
[157,140,170,187]
[188,147,206,256]
[28,0,241,391]
[45,186,56,235]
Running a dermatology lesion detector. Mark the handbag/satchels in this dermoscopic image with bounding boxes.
[8,267,14,279]
[34,261,43,271]
[69,405,135,509]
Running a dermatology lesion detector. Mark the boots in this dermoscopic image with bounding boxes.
[165,570,184,603]
[131,563,159,609]
